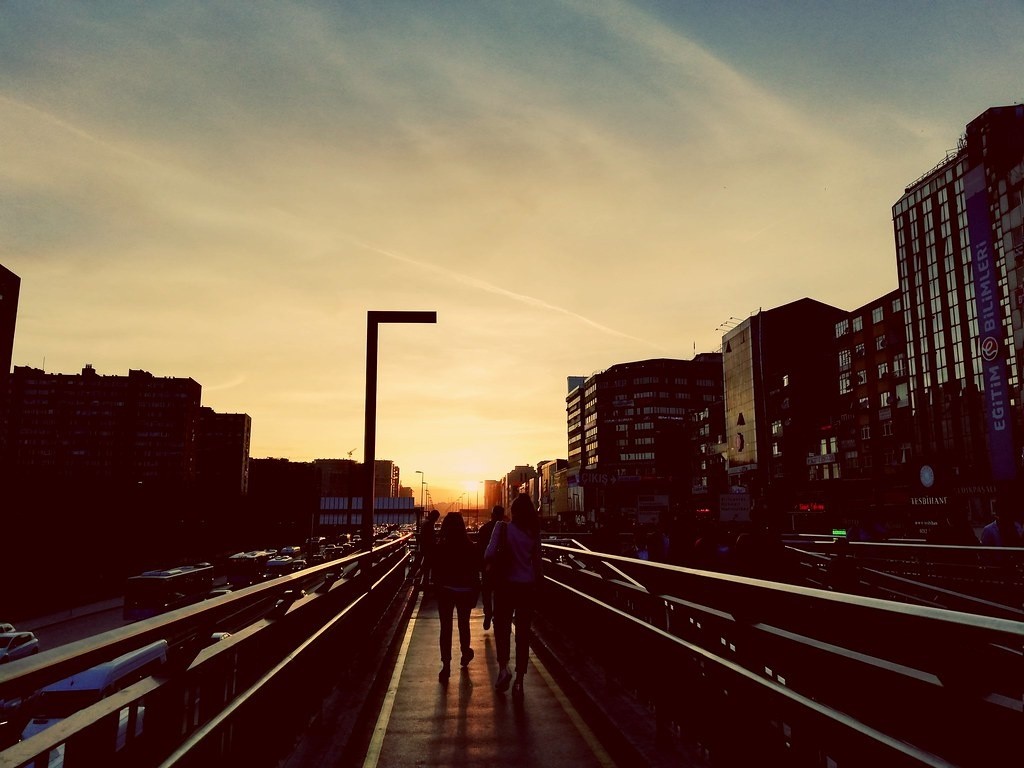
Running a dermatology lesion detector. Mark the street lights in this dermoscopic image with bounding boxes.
[445,480,484,532]
[360,311,440,551]
[415,470,424,528]
[422,481,436,517]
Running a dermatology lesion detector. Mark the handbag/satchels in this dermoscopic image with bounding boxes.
[483,522,508,593]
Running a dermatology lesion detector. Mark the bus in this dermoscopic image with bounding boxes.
[122,560,215,621]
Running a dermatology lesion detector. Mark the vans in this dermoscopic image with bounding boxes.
[16,639,173,768]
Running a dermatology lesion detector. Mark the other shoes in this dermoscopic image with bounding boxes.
[495,665,513,693]
[512,681,524,693]
[439,666,450,678]
[484,611,492,630]
[461,648,474,666]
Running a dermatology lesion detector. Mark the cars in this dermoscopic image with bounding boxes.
[203,517,418,609]
[0,630,39,664]
[1,622,19,635]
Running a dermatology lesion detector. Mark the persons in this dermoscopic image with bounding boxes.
[927,493,1024,597]
[420,494,542,687]
